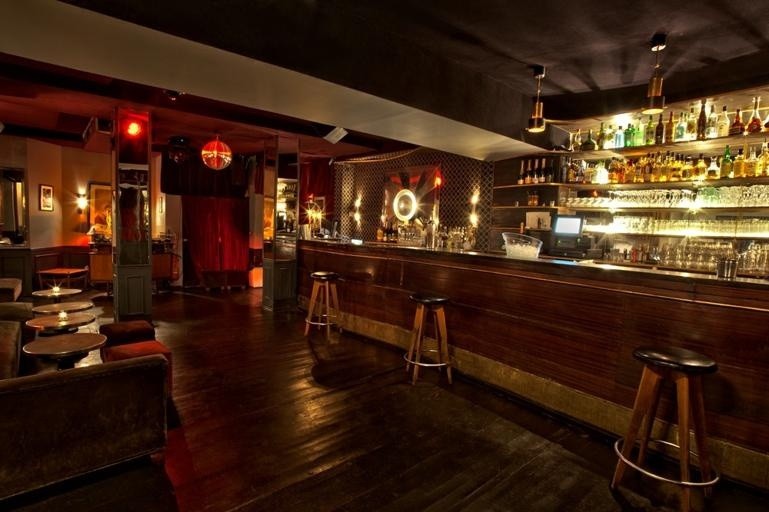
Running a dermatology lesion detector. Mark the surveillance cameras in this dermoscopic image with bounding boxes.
[167,89,179,101]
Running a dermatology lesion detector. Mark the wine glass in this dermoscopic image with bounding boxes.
[607,211,769,276]
[566,184,769,207]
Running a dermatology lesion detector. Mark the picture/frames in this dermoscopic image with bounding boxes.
[88,183,112,228]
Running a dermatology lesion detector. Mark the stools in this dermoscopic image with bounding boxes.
[304,270,343,341]
[607,343,724,511]
[100,339,172,400]
[100,318,156,342]
[403,290,457,388]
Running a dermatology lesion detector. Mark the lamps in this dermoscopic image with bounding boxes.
[200,137,233,171]
[640,33,671,117]
[75,189,88,215]
[523,64,551,136]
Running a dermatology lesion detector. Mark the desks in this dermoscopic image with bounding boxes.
[31,288,83,305]
[21,331,108,370]
[0,277,23,301]
[25,312,96,339]
[31,300,93,318]
[35,265,90,288]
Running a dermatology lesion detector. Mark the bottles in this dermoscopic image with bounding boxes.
[519,222,524,237]
[559,95,768,183]
[516,158,556,183]
[526,189,542,206]
[377,216,474,249]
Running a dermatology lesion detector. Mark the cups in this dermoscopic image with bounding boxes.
[541,202,545,206]
[550,201,555,206]
[513,200,519,207]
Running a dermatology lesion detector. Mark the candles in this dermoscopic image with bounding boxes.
[57,311,68,320]
[52,285,60,293]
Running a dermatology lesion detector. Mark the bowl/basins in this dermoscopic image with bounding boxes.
[502,232,542,259]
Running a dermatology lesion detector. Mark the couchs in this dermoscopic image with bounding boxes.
[0,302,172,506]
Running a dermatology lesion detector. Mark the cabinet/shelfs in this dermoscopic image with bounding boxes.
[88,240,175,298]
[487,154,569,254]
[0,247,33,297]
[562,84,769,278]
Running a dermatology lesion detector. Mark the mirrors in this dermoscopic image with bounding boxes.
[275,136,297,261]
[119,106,148,265]
[0,166,28,244]
[263,134,275,261]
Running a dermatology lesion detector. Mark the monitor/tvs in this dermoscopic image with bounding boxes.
[552,214,584,236]
